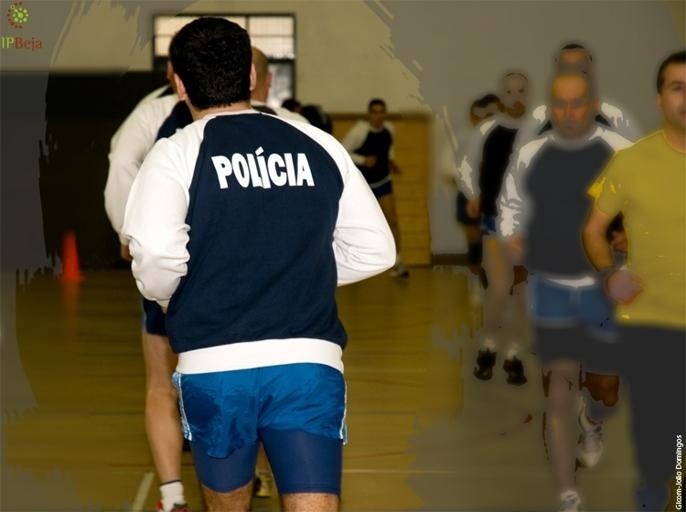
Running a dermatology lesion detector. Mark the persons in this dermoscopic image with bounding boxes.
[248,47,309,499]
[517,40,640,255]
[274,68,543,389]
[499,73,642,512]
[132,15,398,512]
[582,49,686,512]
[102,78,252,512]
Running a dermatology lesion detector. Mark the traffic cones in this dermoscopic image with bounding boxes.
[51,227,82,283]
[61,282,83,337]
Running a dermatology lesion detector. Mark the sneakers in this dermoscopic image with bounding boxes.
[503,356,527,386]
[577,396,605,469]
[473,348,496,381]
